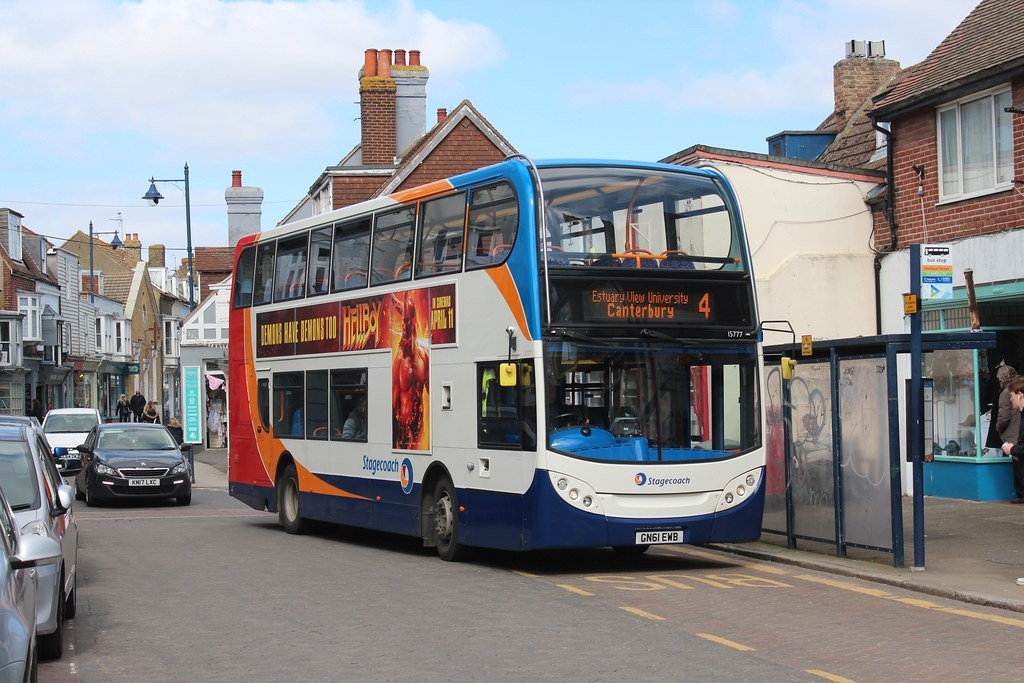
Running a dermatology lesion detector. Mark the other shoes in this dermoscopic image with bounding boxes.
[1017,577,1024,585]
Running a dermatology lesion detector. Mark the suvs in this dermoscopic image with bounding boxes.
[0,415,80,663]
[39,408,103,470]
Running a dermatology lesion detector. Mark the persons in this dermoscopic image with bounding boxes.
[115,390,162,424]
[392,298,429,450]
[343,402,368,439]
[997,364,1024,586]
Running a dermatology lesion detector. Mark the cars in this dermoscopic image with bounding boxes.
[1,484,39,683]
[75,422,194,507]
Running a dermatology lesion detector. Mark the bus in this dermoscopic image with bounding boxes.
[227,151,798,563]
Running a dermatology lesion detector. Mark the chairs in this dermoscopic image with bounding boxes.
[234,245,696,307]
[313,426,341,437]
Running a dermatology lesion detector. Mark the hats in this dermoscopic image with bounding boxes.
[958,414,976,428]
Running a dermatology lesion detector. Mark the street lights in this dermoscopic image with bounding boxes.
[142,160,196,313]
[88,220,123,304]
